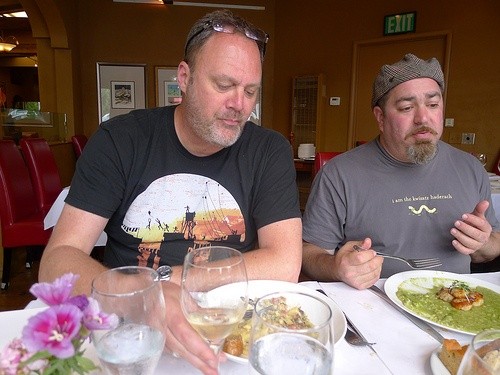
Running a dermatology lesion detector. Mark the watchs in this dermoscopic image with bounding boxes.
[152,265,173,282]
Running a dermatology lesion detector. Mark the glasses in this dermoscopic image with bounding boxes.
[184,19,269,63]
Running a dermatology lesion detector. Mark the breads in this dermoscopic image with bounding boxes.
[223,335,243,357]
[438,337,500,375]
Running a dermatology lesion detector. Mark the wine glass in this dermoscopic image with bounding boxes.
[177,245,248,375]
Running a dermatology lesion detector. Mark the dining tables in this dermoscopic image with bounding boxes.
[0,271,500,375]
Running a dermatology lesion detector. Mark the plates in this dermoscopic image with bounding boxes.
[384,270,500,341]
[200,279,348,369]
[430,338,468,375]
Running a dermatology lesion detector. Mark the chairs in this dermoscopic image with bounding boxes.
[20,137,62,214]
[0,137,54,291]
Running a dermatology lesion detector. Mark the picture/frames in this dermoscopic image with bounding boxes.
[155,67,184,107]
[95,61,147,127]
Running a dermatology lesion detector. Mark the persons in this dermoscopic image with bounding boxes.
[301,54,500,290]
[38,11,303,375]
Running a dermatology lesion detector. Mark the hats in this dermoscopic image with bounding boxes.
[371,53,445,108]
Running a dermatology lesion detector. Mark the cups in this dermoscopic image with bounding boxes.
[468,152,488,166]
[87,263,167,375]
[250,290,339,375]
[467,327,500,375]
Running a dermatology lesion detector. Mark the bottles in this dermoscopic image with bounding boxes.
[298,143,316,158]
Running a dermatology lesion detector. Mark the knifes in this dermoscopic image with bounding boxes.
[368,285,446,345]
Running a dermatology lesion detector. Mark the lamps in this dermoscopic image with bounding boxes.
[0,32,20,52]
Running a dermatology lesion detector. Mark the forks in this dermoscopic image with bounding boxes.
[353,245,443,268]
[314,288,377,347]
